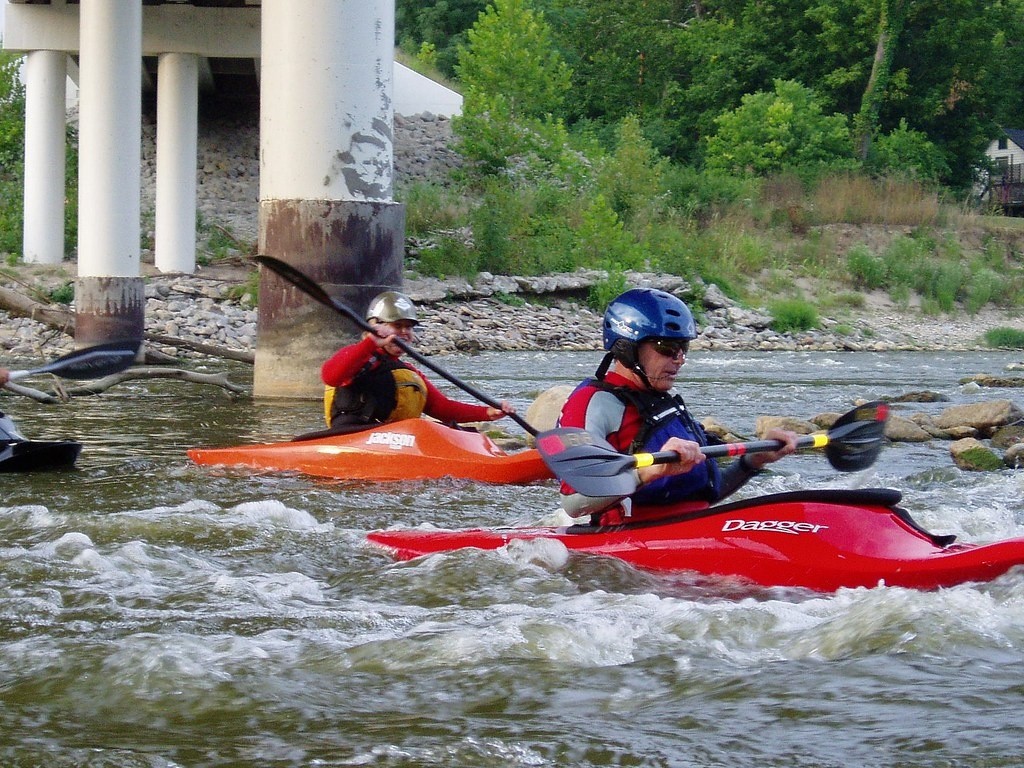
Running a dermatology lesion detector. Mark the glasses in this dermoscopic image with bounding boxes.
[647,337,690,357]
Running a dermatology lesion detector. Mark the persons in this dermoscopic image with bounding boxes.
[554,289,799,526]
[320,289,517,431]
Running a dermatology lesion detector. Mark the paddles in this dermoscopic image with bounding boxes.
[0,343,135,384]
[247,253,539,434]
[534,398,890,498]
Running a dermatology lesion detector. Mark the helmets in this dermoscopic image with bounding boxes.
[602,288,698,368]
[365,290,418,324]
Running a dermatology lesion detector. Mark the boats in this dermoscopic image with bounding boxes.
[358,487,1024,596]
[0,409,86,471]
[184,415,559,485]
